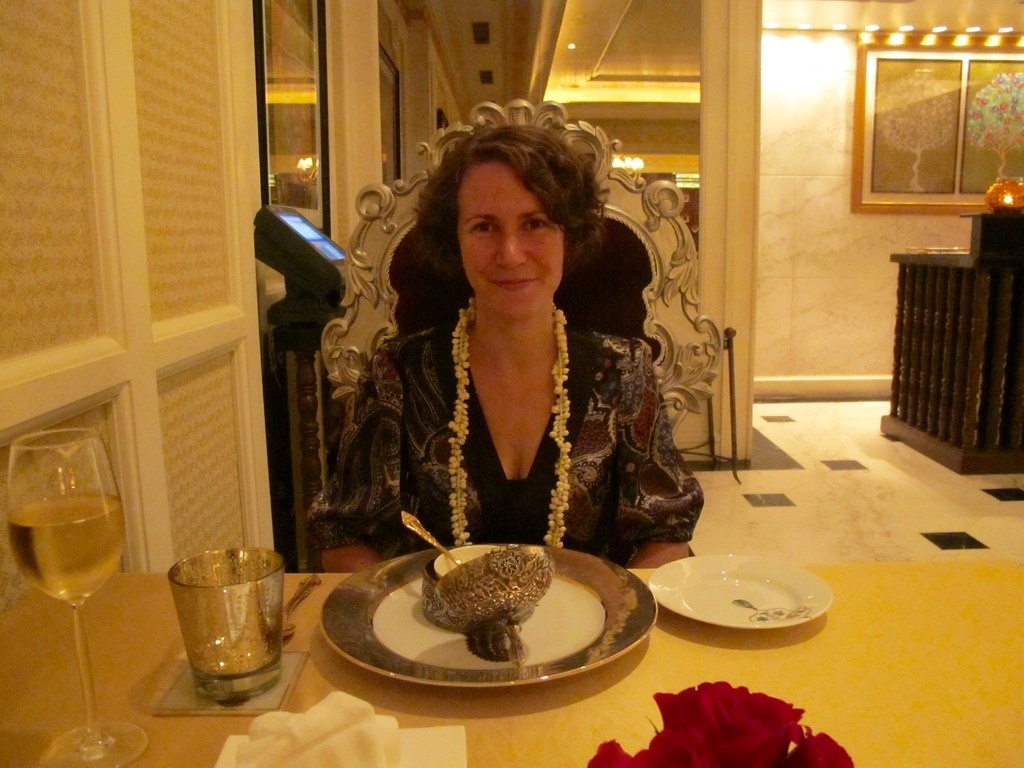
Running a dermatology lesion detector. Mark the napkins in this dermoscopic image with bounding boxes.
[234,689,402,768]
[215,723,468,768]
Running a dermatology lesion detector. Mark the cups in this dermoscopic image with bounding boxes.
[167,547,285,700]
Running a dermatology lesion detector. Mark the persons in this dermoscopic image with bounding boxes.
[311,118,708,575]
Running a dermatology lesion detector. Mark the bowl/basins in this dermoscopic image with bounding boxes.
[434,545,501,578]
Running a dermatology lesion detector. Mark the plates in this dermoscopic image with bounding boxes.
[648,554,834,629]
[321,543,657,687]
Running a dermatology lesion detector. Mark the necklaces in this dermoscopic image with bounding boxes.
[446,297,573,555]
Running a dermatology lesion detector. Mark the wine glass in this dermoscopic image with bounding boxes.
[7,429,149,767]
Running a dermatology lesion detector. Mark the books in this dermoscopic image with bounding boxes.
[907,245,969,256]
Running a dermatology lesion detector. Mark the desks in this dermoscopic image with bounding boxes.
[0,554,1024,768]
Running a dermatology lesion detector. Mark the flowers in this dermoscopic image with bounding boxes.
[584,681,857,768]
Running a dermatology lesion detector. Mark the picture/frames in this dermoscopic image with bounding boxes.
[849,32,1024,217]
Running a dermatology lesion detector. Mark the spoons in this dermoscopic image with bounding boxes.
[268,574,321,638]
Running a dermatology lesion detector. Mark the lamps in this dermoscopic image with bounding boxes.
[986,176,1024,215]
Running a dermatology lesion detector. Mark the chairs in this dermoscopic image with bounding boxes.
[316,99,726,578]
